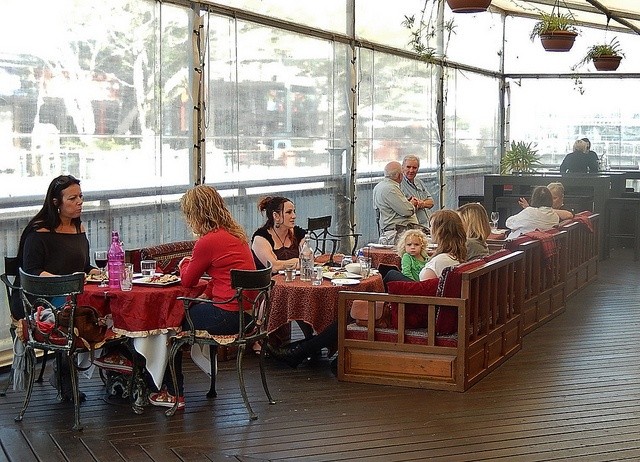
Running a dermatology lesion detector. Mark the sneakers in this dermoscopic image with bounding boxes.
[49,370,86,401]
[90,350,134,374]
[148,390,187,410]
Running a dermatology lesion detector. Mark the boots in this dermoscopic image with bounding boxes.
[265,340,305,368]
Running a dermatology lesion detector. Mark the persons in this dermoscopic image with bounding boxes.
[501,186,560,243]
[560,140,588,174]
[455,203,491,262]
[148,185,262,410]
[373,161,419,244]
[250,195,338,368]
[266,210,467,369]
[397,229,431,281]
[11,175,168,398]
[400,154,435,228]
[519,182,575,223]
[581,138,601,172]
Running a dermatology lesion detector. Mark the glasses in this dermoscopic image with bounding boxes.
[53,175,76,189]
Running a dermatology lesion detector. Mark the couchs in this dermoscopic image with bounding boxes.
[551,209,602,301]
[336,248,526,394]
[501,228,568,338]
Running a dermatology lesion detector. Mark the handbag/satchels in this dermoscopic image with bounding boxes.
[394,223,430,245]
[56,305,107,343]
[350,300,393,329]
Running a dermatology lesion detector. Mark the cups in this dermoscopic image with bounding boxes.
[140,260,157,277]
[284,263,296,283]
[312,266,323,285]
[359,257,371,280]
[118,262,133,291]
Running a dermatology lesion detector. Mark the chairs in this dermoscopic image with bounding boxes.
[164,259,277,421]
[0,255,47,397]
[13,267,118,432]
[304,213,363,264]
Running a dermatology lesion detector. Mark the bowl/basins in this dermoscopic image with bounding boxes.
[345,262,360,274]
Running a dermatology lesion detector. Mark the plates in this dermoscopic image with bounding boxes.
[132,275,182,287]
[322,271,363,279]
[368,242,394,249]
[426,243,439,248]
[85,279,110,282]
[331,279,361,285]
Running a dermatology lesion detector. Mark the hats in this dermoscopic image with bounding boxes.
[575,140,587,152]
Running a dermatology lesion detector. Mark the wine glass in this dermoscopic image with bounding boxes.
[491,211,499,232]
[95,250,110,288]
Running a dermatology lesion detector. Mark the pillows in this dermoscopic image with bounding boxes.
[385,279,440,331]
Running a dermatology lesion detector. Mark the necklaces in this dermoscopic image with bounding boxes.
[271,226,289,250]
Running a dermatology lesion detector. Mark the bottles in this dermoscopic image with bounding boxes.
[108,231,124,288]
[299,234,315,282]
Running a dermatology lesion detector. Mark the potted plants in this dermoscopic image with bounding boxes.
[400,0,494,78]
[529,12,579,51]
[570,36,627,94]
[498,139,540,176]
